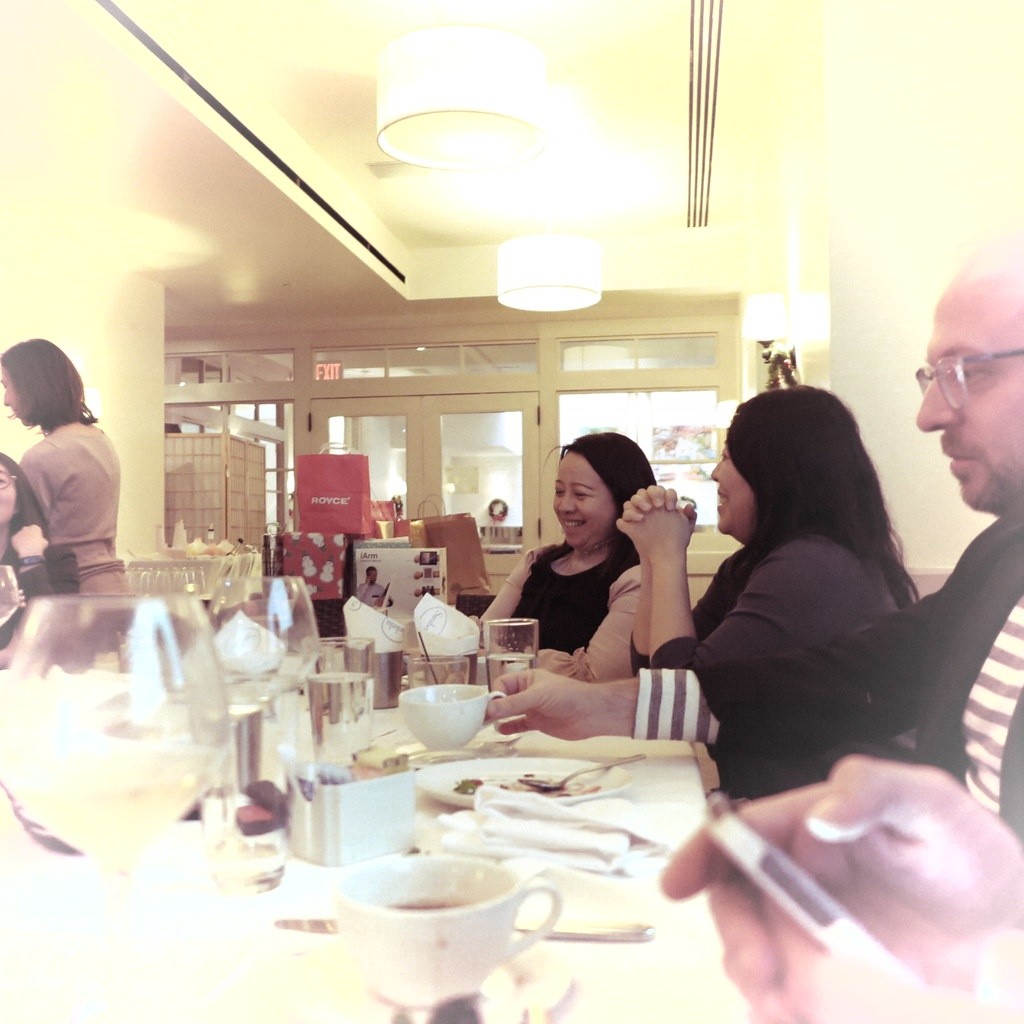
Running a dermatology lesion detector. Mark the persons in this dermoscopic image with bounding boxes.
[472,432,657,682]
[0,452,82,653]
[660,759,1024,1024]
[0,338,128,594]
[487,236,1024,838]
[616,386,919,676]
[357,566,394,608]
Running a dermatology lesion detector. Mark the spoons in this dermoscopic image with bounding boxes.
[518,752,647,792]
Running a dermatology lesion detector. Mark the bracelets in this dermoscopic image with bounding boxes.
[17,555,46,566]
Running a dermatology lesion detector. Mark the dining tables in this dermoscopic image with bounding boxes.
[1,710,755,1024]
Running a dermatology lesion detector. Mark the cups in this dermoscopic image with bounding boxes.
[372,650,403,710]
[301,636,378,767]
[0,564,19,627]
[200,670,308,897]
[331,852,563,1009]
[398,684,516,753]
[484,617,539,695]
[407,656,470,689]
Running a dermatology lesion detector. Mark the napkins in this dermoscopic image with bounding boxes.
[413,591,480,656]
[437,784,664,878]
[343,596,406,653]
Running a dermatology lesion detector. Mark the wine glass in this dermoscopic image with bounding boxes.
[206,575,321,696]
[1,589,232,1024]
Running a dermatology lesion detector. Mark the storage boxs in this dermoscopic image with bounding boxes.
[281,531,346,598]
[354,547,449,647]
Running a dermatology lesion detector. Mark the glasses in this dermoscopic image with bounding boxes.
[0,470,19,490]
[914,349,1024,410]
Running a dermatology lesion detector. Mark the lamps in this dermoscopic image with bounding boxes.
[374,27,542,176]
[746,292,830,388]
[497,234,602,312]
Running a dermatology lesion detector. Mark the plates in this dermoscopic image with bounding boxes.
[414,756,635,811]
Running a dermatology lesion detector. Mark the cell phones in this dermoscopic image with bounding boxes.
[711,814,915,983]
